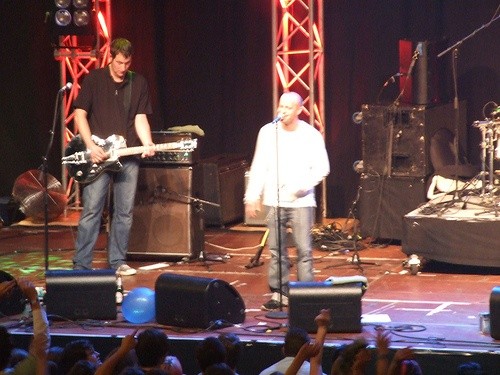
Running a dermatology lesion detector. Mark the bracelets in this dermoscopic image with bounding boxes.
[30,298,39,305]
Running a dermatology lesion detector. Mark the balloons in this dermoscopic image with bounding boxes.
[121,287,155,324]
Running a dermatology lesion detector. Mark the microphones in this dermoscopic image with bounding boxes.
[156,185,168,193]
[273,114,283,124]
[57,82,73,94]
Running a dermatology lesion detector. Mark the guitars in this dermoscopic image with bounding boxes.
[62,133,199,185]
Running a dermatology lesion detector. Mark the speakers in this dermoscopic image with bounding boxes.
[488,285,500,341]
[357,100,468,239]
[44,269,117,320]
[199,153,251,228]
[109,162,204,260]
[0,270,25,317]
[288,281,362,334]
[155,272,246,330]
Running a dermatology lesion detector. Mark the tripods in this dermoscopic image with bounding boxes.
[433,16,500,217]
[324,193,382,274]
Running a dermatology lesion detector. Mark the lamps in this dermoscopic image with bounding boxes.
[49,0,90,35]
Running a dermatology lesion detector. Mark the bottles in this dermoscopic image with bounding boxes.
[479,312,490,336]
[115,275,124,305]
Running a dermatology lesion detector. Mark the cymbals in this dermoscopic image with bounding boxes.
[12,169,68,223]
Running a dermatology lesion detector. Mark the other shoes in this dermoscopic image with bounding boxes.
[114,263,137,276]
[260,299,282,311]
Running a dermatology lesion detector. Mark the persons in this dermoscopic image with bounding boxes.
[243,92,330,311]
[72,38,156,275]
[0,276,422,375]
[458,362,481,375]
